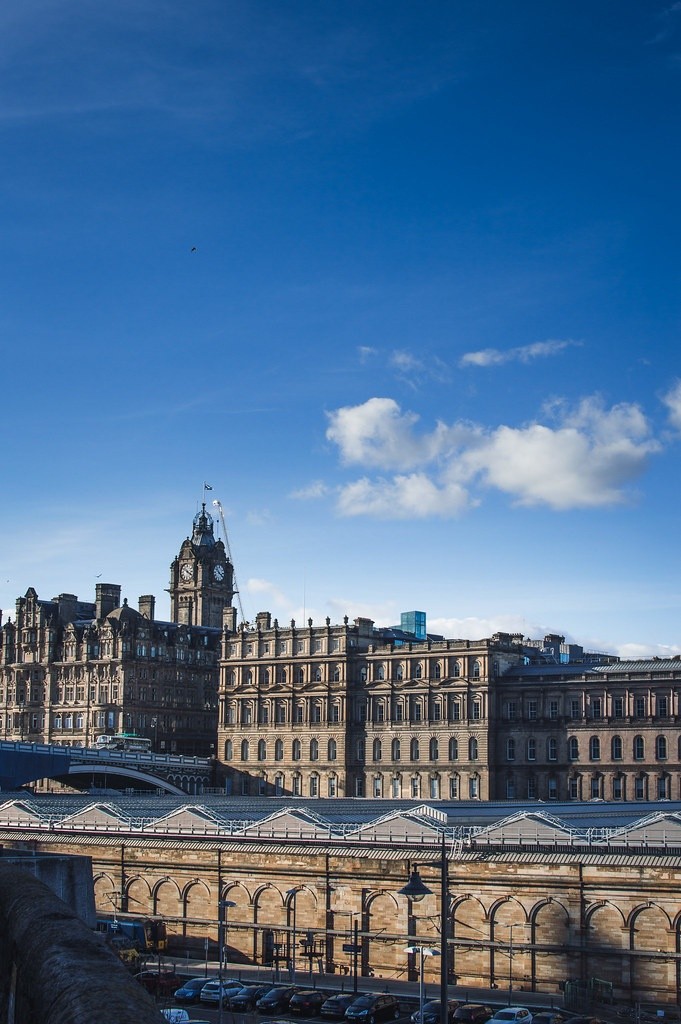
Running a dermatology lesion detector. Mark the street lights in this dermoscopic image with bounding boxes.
[151,717,157,754]
[503,923,520,993]
[286,889,302,983]
[399,859,448,1024]
[217,901,234,977]
[19,701,25,742]
[402,946,440,1024]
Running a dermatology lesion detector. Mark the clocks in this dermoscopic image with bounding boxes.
[212,563,225,582]
[180,563,194,581]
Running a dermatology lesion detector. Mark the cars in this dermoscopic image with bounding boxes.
[587,797,603,802]
[173,977,599,1024]
[133,969,178,994]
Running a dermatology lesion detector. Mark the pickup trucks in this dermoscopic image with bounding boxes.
[160,1008,210,1024]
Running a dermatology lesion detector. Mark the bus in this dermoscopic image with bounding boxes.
[95,733,152,754]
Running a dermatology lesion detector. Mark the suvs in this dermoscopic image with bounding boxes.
[485,1007,533,1024]
[200,980,246,1004]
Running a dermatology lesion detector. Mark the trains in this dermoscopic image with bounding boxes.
[94,930,141,964]
[95,918,167,954]
